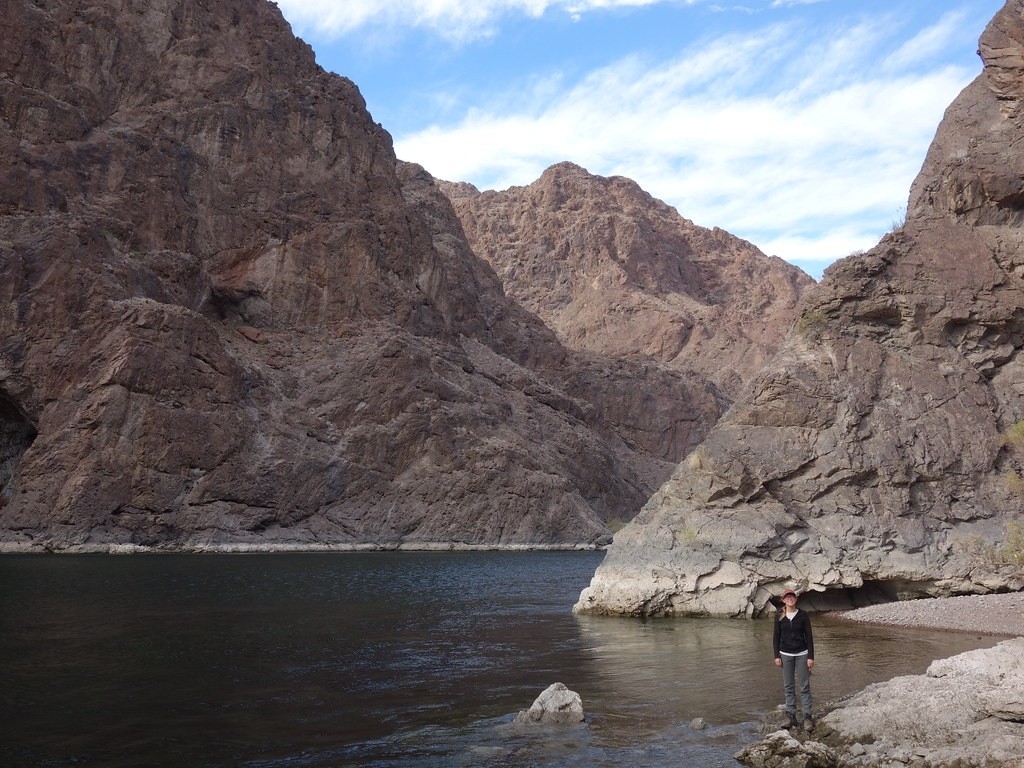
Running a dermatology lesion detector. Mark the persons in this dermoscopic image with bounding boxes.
[773,590,814,731]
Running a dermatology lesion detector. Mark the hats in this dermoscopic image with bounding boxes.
[782,588,796,598]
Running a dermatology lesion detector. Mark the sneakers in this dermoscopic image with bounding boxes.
[781,712,799,728]
[804,715,813,731]
